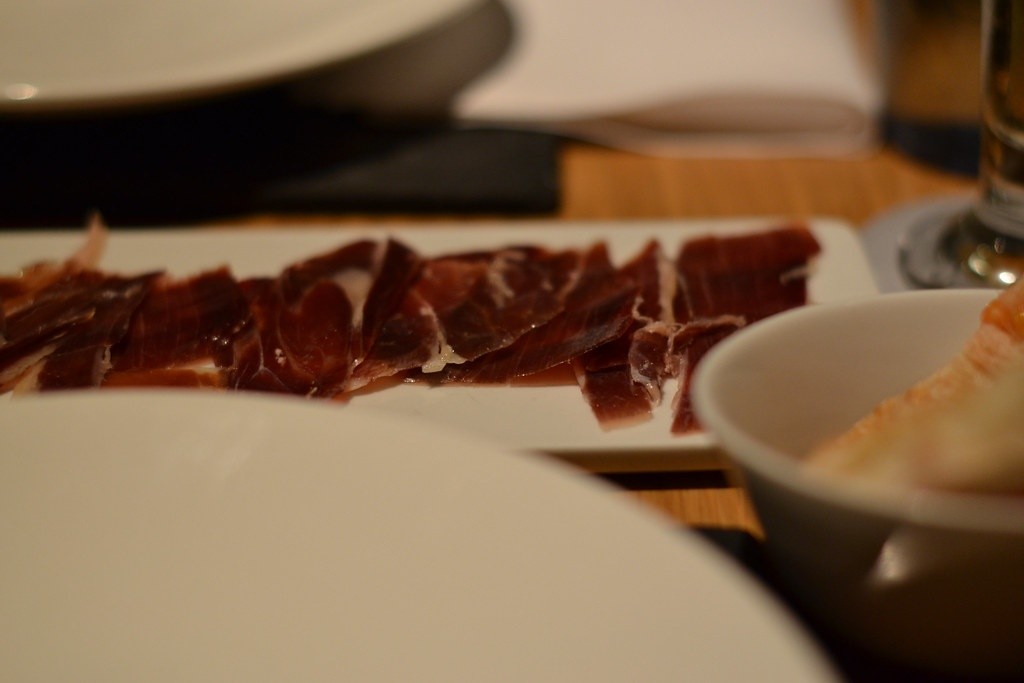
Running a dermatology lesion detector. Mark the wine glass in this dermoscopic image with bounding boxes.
[901,0,1024,292]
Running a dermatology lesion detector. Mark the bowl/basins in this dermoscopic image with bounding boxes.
[690,281,1023,683]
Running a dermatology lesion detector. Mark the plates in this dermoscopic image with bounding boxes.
[0,386,836,683]
[0,215,878,472]
